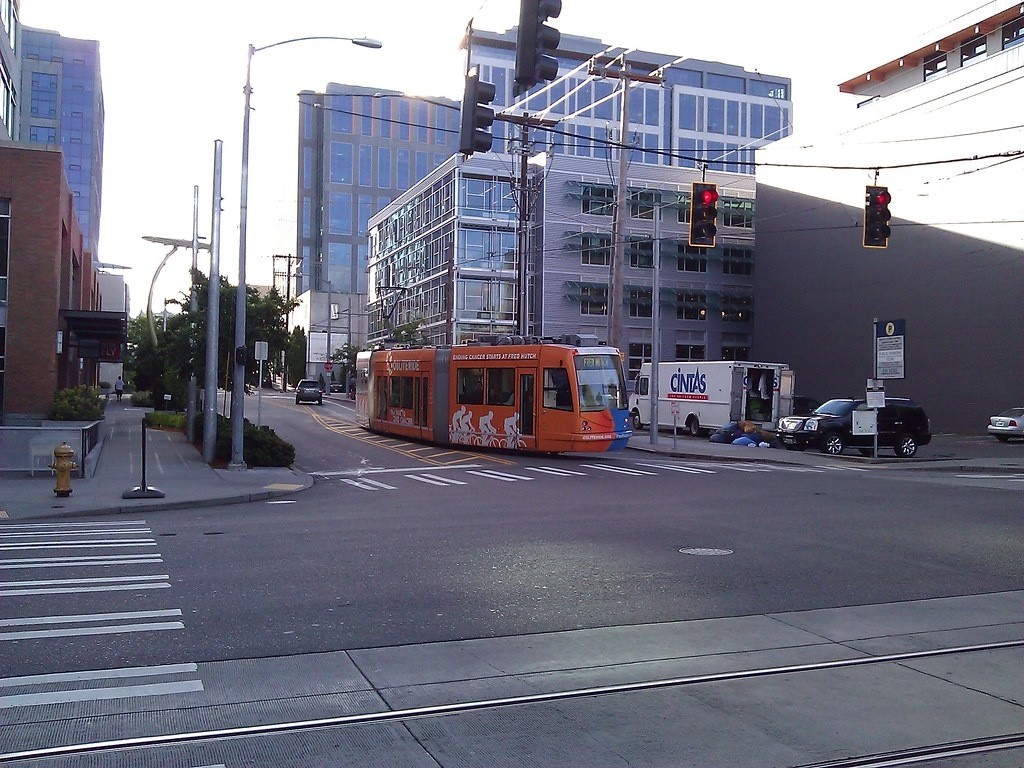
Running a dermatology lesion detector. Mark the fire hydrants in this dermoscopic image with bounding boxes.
[48,441,78,498]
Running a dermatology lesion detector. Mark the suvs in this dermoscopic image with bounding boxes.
[294,379,323,405]
[776,397,932,458]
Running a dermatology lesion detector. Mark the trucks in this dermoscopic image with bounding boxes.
[628,361,794,438]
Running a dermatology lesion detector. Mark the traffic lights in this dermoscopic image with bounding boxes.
[459,64,496,154]
[689,182,717,246]
[864,186,891,249]
[513,1,561,98]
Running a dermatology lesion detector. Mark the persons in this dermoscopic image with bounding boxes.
[114,375,125,403]
[470,380,483,404]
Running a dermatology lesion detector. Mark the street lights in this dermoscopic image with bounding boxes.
[228,33,382,476]
[650,202,687,451]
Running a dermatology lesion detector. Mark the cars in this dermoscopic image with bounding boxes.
[987,407,1024,442]
[350,388,356,399]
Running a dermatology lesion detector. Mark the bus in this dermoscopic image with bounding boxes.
[355,333,633,457]
